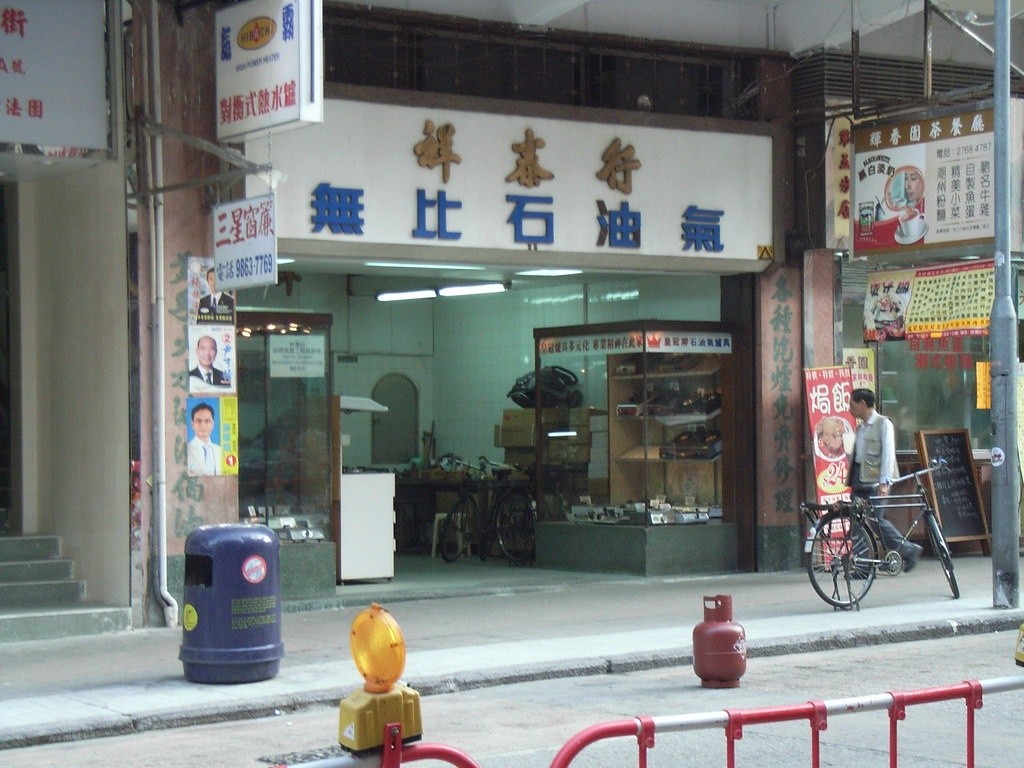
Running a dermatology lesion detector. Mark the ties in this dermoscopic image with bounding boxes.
[211,297,216,313]
[206,372,212,384]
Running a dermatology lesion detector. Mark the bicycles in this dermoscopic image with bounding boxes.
[797,456,960,612]
[436,456,540,567]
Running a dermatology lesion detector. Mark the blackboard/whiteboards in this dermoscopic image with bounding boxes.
[920,429,990,542]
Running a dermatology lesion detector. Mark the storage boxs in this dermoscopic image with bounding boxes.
[487,407,609,558]
[415,469,465,482]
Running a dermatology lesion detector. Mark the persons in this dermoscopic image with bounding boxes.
[902,168,923,204]
[196,268,234,323]
[188,402,222,477]
[845,389,924,579]
[190,335,231,385]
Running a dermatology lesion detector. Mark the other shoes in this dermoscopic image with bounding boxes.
[903,544,922,572]
[844,572,875,579]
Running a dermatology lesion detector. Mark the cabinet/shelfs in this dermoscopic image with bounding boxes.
[339,473,396,586]
[606,351,721,506]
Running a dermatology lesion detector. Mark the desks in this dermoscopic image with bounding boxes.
[396,478,528,557]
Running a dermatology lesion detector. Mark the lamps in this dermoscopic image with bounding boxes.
[375,288,438,302]
[437,280,513,297]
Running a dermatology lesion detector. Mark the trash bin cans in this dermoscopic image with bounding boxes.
[178,523,284,686]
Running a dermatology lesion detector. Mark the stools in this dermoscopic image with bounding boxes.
[431,512,471,558]
